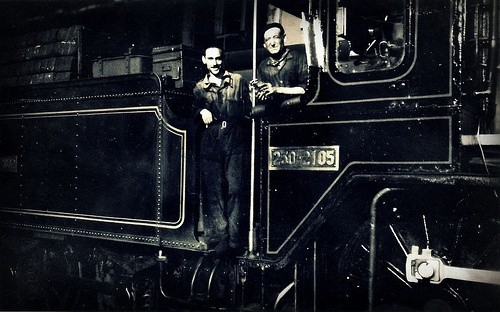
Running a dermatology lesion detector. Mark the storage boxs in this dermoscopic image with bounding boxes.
[93,44,210,88]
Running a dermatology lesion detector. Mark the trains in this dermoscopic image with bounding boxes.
[0,0,500,312]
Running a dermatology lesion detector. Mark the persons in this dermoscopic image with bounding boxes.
[250,23,309,101]
[190,45,251,254]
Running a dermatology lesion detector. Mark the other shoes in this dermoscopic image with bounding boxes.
[228,239,238,248]
[209,233,229,244]
